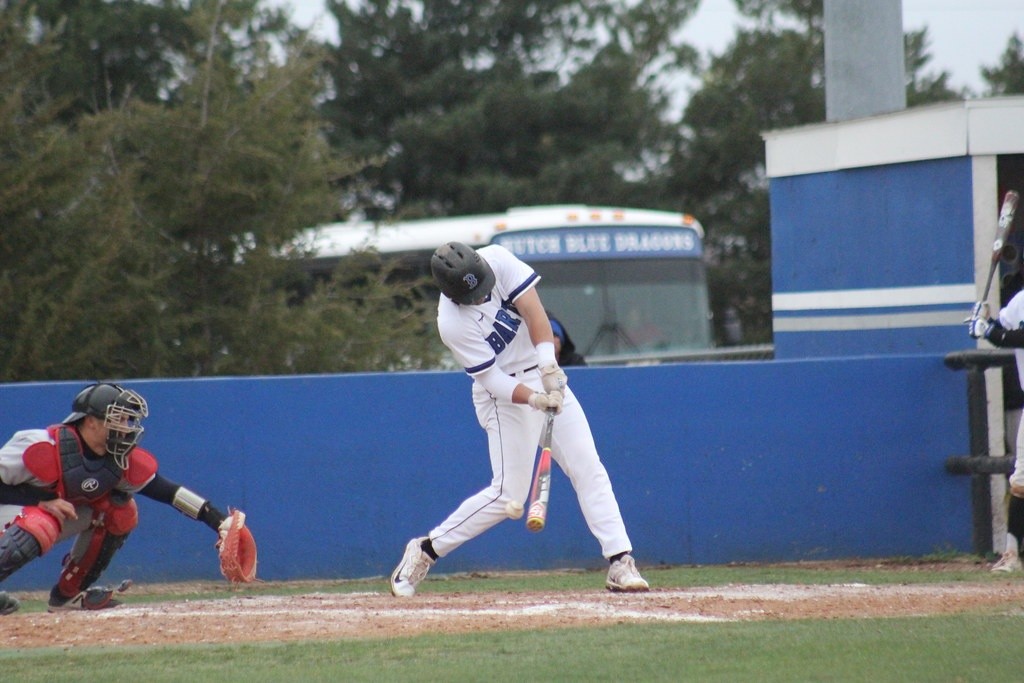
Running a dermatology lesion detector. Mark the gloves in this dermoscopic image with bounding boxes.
[962,315,995,337]
[540,363,568,398]
[528,391,564,417]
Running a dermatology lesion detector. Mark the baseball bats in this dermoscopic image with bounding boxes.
[969,189,1018,342]
[526,412,555,535]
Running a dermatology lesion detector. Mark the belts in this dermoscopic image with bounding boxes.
[511,365,539,376]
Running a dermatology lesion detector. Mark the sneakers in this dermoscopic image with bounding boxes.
[989,552,1023,574]
[389,535,441,597]
[604,556,649,592]
[46,589,113,613]
[0,591,20,614]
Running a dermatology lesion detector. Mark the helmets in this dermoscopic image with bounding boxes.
[431,241,495,304]
[62,382,138,424]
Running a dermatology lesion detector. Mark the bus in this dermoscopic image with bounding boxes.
[229,204,742,376]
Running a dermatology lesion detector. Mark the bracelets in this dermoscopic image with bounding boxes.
[535,342,556,362]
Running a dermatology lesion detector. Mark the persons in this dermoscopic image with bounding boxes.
[391,240,650,593]
[546,310,587,367]
[968,286,1024,574]
[0,383,256,613]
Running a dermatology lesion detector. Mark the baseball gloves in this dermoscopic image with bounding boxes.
[220,513,257,581]
